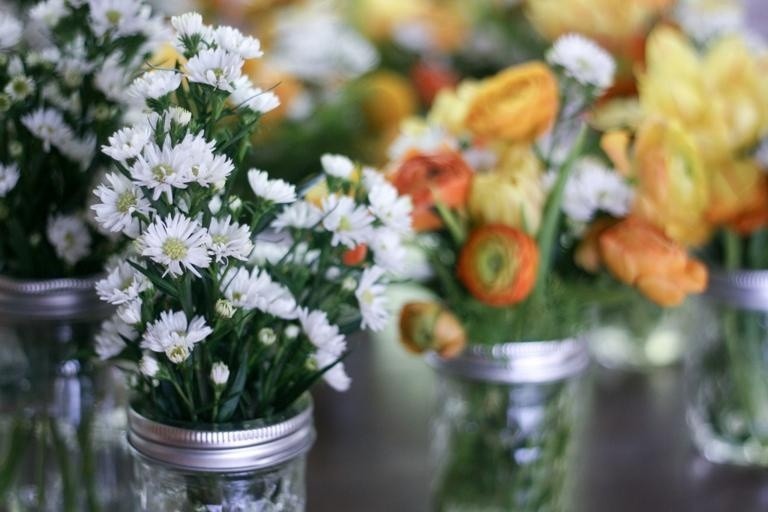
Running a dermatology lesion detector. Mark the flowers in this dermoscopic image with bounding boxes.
[0,1,767,509]
